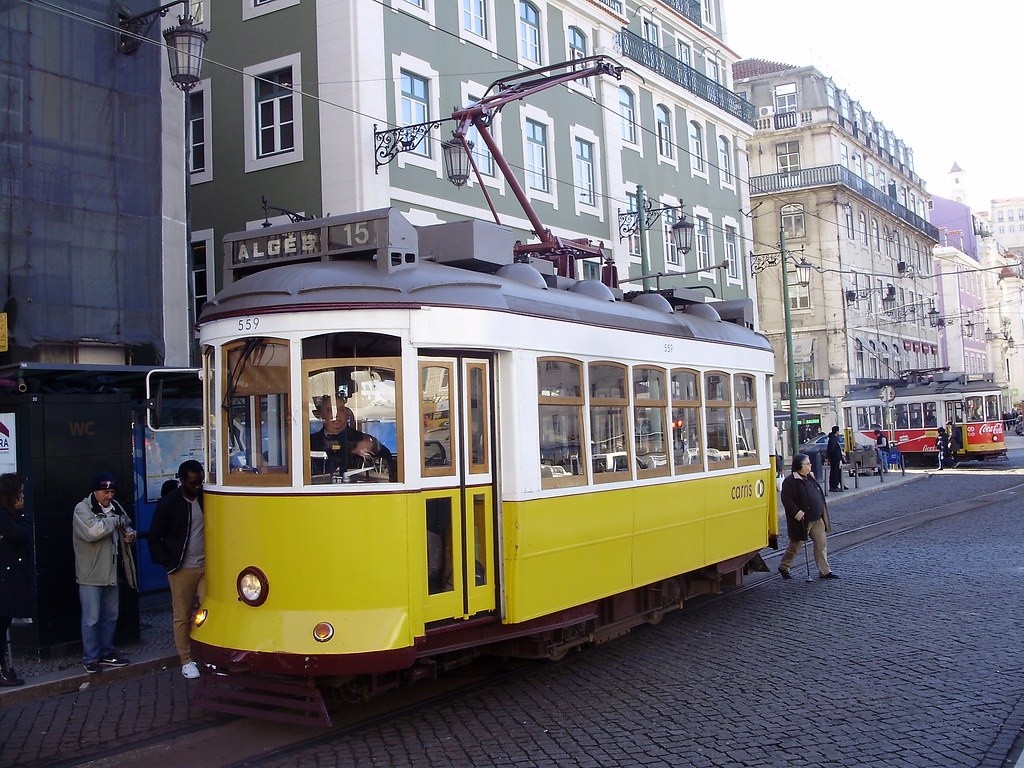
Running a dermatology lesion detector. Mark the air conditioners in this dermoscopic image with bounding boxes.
[758,106,773,117]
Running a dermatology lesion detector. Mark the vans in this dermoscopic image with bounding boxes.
[591,451,646,473]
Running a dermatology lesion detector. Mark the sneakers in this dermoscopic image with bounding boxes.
[84,661,99,674]
[182,663,200,679]
[98,657,129,667]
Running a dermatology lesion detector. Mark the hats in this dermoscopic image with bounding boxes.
[946,421,952,425]
[92,473,115,490]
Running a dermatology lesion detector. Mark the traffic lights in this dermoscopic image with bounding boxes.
[889,408,899,425]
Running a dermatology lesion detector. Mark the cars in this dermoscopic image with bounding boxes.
[540,464,572,478]
[649,445,725,469]
[799,433,844,464]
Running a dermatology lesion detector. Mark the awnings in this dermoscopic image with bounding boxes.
[774,410,815,420]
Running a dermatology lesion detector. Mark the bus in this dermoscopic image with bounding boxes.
[773,409,821,445]
[189,55,780,729]
[840,365,1009,470]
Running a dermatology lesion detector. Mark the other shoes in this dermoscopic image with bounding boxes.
[777,565,792,579]
[820,572,839,580]
[0,671,25,686]
[828,487,842,492]
[841,485,850,490]
[953,461,960,469]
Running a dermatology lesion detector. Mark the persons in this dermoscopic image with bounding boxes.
[779,453,840,578]
[0,472,32,685]
[150,459,208,677]
[815,428,827,443]
[827,426,849,492]
[1002,408,1022,431]
[937,420,963,469]
[935,427,958,470]
[874,429,891,473]
[72,473,141,672]
[310,394,392,477]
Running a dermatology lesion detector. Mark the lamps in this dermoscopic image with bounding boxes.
[618,198,695,257]
[846,284,896,316]
[937,316,975,337]
[119,0,210,92]
[374,105,476,190]
[898,300,940,327]
[261,194,312,228]
[992,330,1015,349]
[961,319,993,343]
[750,244,813,288]
